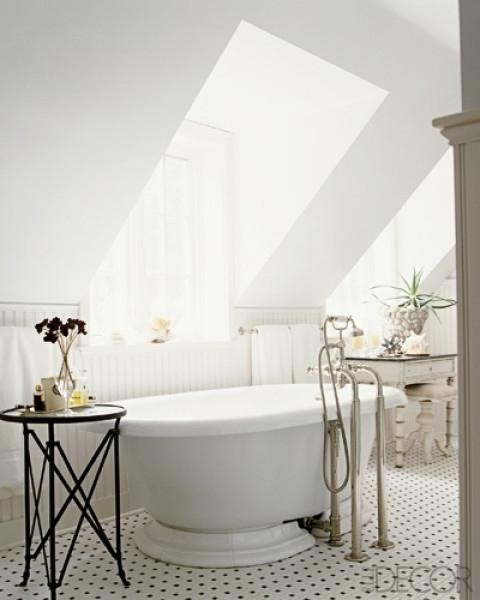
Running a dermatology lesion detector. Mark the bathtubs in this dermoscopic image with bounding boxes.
[81,384,407,531]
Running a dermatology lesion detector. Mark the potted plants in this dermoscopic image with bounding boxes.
[369,263,459,338]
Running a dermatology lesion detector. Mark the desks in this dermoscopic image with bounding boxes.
[1,403,128,600]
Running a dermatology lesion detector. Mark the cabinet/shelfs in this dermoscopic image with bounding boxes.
[344,352,458,469]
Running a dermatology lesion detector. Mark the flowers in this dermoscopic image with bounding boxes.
[33,312,88,387]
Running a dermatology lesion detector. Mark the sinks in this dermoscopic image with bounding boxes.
[376,353,402,359]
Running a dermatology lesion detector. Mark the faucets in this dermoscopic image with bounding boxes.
[390,333,405,348]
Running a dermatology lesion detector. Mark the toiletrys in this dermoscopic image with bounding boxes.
[70,384,97,406]
[33,385,46,411]
